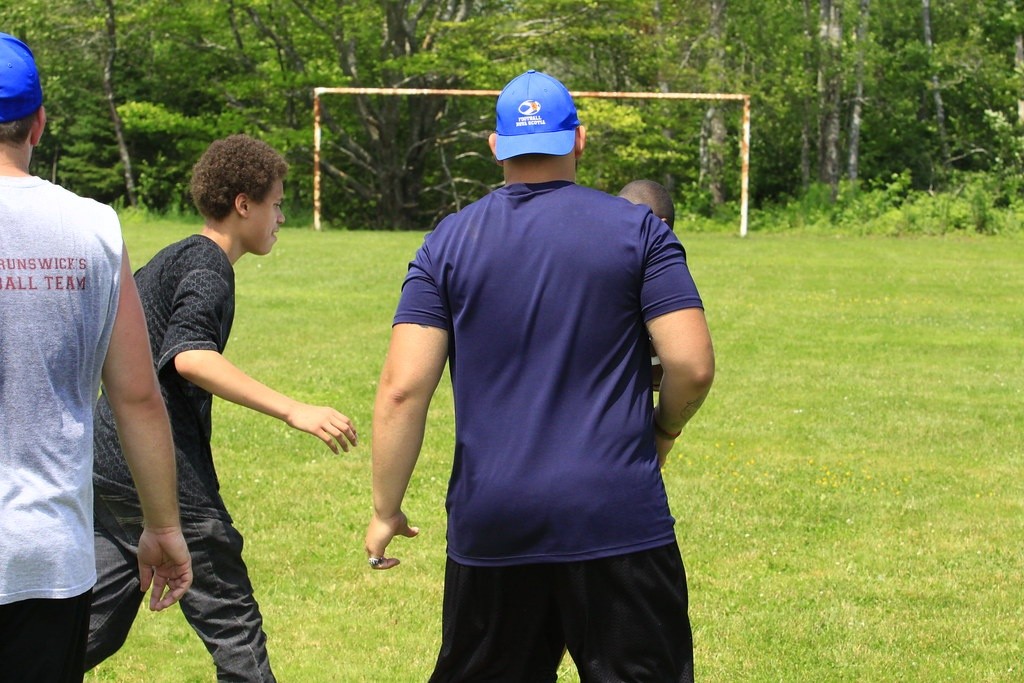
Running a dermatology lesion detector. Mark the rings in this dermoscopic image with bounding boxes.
[367,556,383,566]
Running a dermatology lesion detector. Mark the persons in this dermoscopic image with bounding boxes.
[81,134,358,682]
[363,68,717,682]
[1,31,195,683]
[614,179,676,393]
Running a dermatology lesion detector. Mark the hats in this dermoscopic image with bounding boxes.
[0,31,43,122]
[496,69,579,161]
